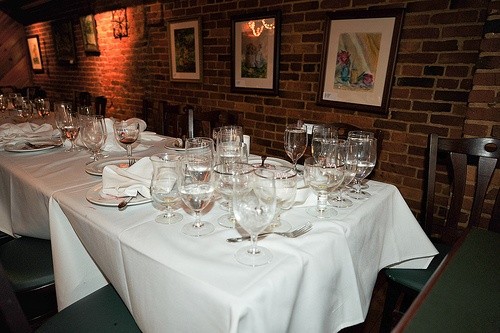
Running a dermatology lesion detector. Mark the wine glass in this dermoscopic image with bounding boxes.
[149,126,296,267]
[283,124,308,176]
[113,122,142,157]
[53,102,92,153]
[0,93,46,123]
[304,131,377,219]
[78,113,107,161]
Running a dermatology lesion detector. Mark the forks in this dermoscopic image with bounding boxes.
[129,158,135,166]
[25,142,58,148]
[226,223,312,242]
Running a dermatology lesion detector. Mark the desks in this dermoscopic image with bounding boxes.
[0,108,439,333]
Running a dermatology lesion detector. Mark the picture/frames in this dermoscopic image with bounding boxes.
[79,10,99,54]
[167,15,203,83]
[316,5,406,114]
[50,17,77,67]
[230,9,281,95]
[25,35,44,74]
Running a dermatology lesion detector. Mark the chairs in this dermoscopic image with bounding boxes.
[378,133,500,333]
[0,84,221,140]
[0,253,140,333]
[0,237,54,325]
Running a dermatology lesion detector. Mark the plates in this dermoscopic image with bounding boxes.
[5,142,62,152]
[249,154,292,172]
[87,182,153,206]
[163,143,210,154]
[85,155,142,175]
